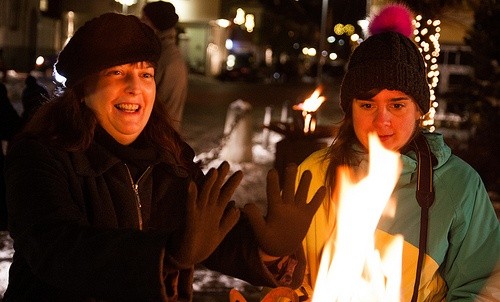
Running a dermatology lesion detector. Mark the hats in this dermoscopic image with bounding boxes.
[57,13,163,87]
[340,30,430,115]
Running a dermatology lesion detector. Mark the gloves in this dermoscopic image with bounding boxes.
[176,161,243,267]
[245,161,327,256]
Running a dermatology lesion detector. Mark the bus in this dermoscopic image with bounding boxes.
[437,46,479,95]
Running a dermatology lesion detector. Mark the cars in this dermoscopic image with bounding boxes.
[214,52,259,81]
